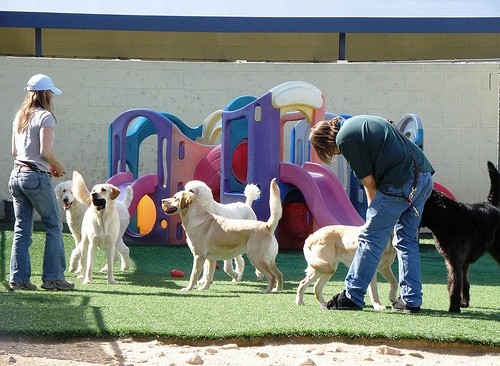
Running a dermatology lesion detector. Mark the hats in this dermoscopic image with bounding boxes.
[24,74,62,95]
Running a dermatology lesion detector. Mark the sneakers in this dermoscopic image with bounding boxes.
[9,280,36,290]
[42,277,74,290]
[391,297,420,313]
[327,290,362,310]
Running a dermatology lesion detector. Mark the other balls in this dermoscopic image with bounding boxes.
[91,199,107,206]
[49,164,61,177]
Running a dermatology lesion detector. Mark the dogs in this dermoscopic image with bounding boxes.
[418,161,499,313]
[161,177,283,294]
[184,180,265,284]
[72,170,122,286]
[53,180,135,280]
[294,221,399,312]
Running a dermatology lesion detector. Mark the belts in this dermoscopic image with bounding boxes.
[15,164,37,170]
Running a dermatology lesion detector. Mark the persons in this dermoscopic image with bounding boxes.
[8,73,75,291]
[308,114,435,314]
